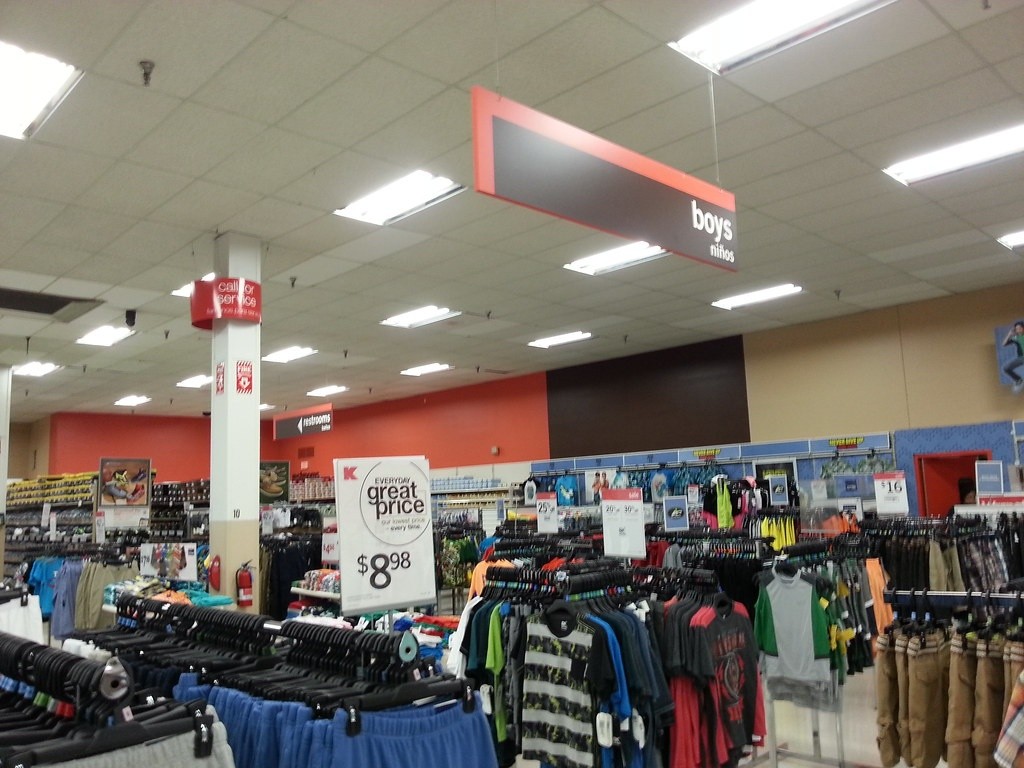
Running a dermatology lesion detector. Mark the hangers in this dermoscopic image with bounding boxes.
[5,527,321,573]
[0,630,218,767]
[60,595,476,737]
[432,506,1024,654]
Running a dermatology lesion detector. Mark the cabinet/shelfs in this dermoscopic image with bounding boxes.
[6,484,337,542]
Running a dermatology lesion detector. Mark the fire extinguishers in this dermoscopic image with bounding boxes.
[234,559,257,607]
